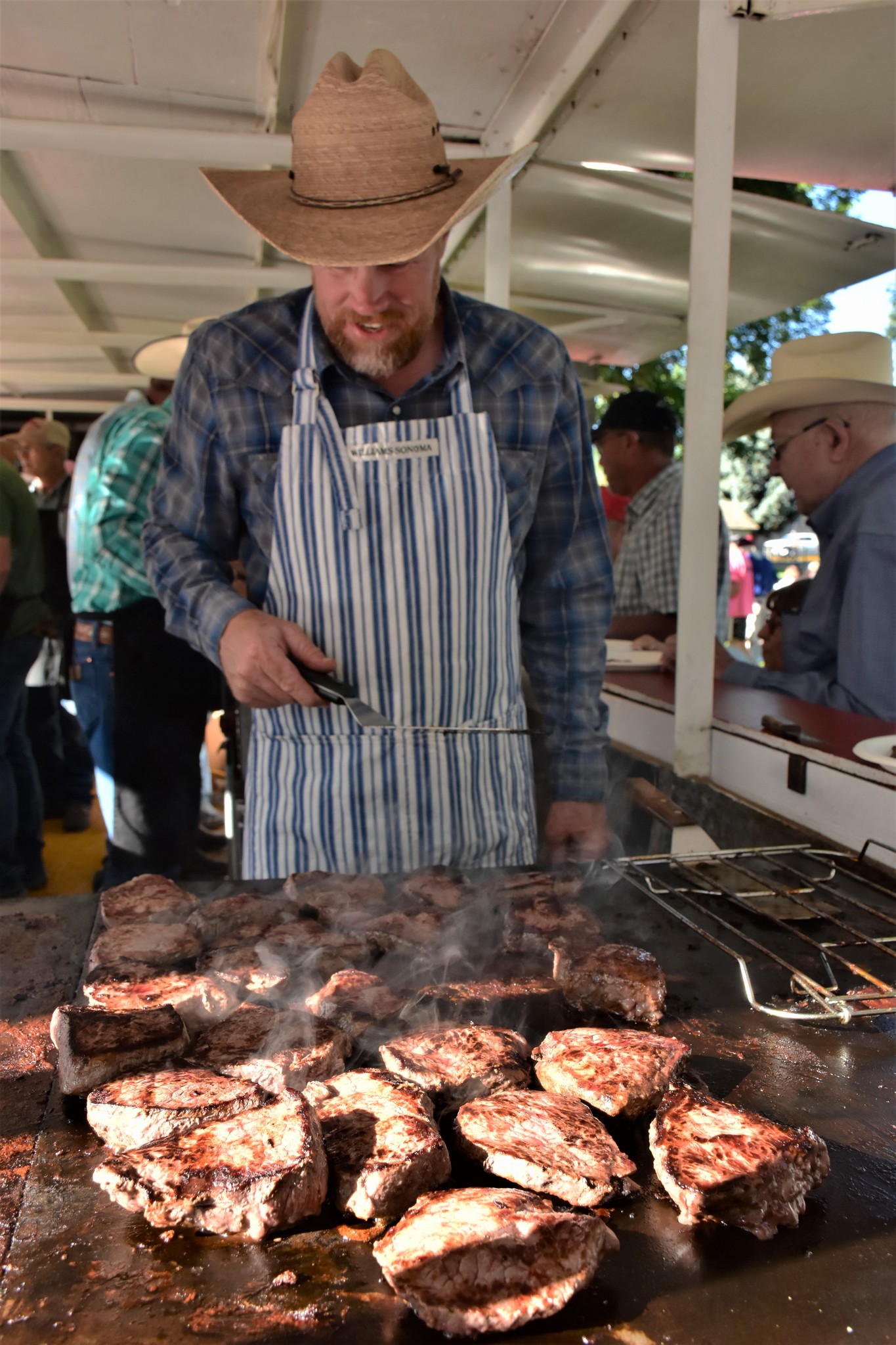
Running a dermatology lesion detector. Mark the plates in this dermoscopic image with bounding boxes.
[604,638,665,673]
[852,736,896,779]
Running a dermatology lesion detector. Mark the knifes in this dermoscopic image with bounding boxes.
[761,714,819,741]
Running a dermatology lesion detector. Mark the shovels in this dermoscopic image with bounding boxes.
[302,668,529,733]
[622,775,849,920]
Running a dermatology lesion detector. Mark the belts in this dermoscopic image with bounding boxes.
[73,622,113,645]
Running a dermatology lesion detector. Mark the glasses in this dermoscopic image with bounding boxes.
[764,619,781,634]
[767,416,850,461]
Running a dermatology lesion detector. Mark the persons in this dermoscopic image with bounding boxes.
[728,542,754,641]
[0,417,96,897]
[738,533,780,661]
[598,486,632,564]
[139,47,614,879]
[588,389,732,645]
[657,329,896,721]
[626,577,817,672]
[72,313,227,887]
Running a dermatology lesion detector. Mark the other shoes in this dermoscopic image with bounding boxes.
[200,796,225,831]
[185,849,228,879]
[198,829,227,852]
[62,803,91,832]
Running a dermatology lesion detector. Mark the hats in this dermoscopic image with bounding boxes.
[589,390,677,442]
[133,335,190,382]
[0,418,72,449]
[199,48,541,268]
[722,332,896,444]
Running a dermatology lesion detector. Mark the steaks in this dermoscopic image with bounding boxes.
[51,860,839,1333]
